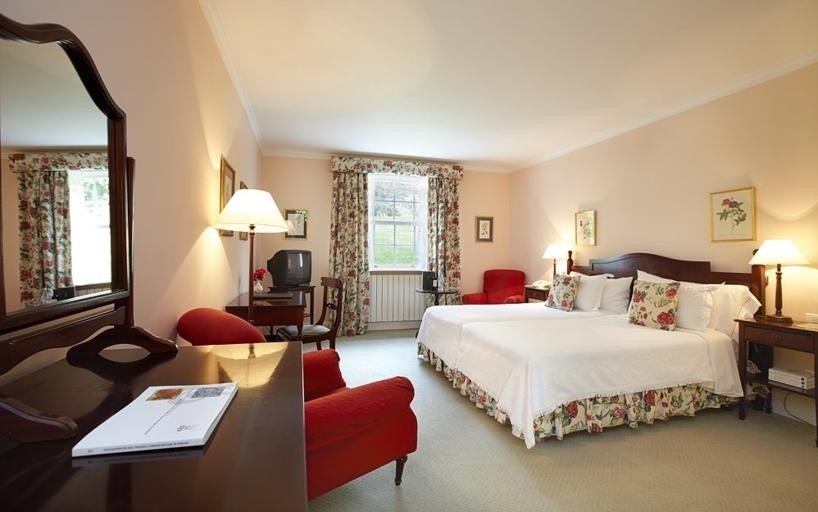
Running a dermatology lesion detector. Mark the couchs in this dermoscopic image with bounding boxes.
[462,269,526,304]
[178,307,418,501]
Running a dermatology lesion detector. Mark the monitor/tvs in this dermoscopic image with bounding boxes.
[267,249,312,287]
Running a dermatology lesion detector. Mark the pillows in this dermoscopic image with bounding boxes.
[635,269,725,334]
[600,277,633,313]
[544,273,581,312]
[710,284,751,337]
[626,280,680,332]
[569,271,614,313]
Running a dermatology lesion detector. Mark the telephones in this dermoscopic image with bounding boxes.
[532,280,550,288]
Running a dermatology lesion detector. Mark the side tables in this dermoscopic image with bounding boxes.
[415,288,458,337]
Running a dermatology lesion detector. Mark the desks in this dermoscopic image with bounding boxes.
[0,340,309,512]
[267,284,316,336]
[225,290,307,342]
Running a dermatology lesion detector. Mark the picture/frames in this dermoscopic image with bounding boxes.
[219,156,235,237]
[474,215,494,242]
[574,210,597,247]
[283,209,310,241]
[239,180,249,240]
[707,186,756,243]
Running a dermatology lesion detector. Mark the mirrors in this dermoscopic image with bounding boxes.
[0,15,179,442]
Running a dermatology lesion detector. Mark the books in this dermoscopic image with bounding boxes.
[72,382,240,457]
[767,365,815,390]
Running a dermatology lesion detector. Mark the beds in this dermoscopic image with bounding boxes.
[416,248,768,450]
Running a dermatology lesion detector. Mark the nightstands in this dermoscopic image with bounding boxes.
[733,316,818,447]
[524,283,550,303]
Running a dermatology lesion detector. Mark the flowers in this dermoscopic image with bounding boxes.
[254,266,270,282]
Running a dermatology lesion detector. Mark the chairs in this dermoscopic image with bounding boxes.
[276,276,344,351]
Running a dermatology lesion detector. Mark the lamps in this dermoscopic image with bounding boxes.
[212,188,288,326]
[541,245,565,281]
[748,237,810,325]
[211,342,288,389]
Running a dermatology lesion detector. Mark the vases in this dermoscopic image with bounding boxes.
[254,279,263,293]
